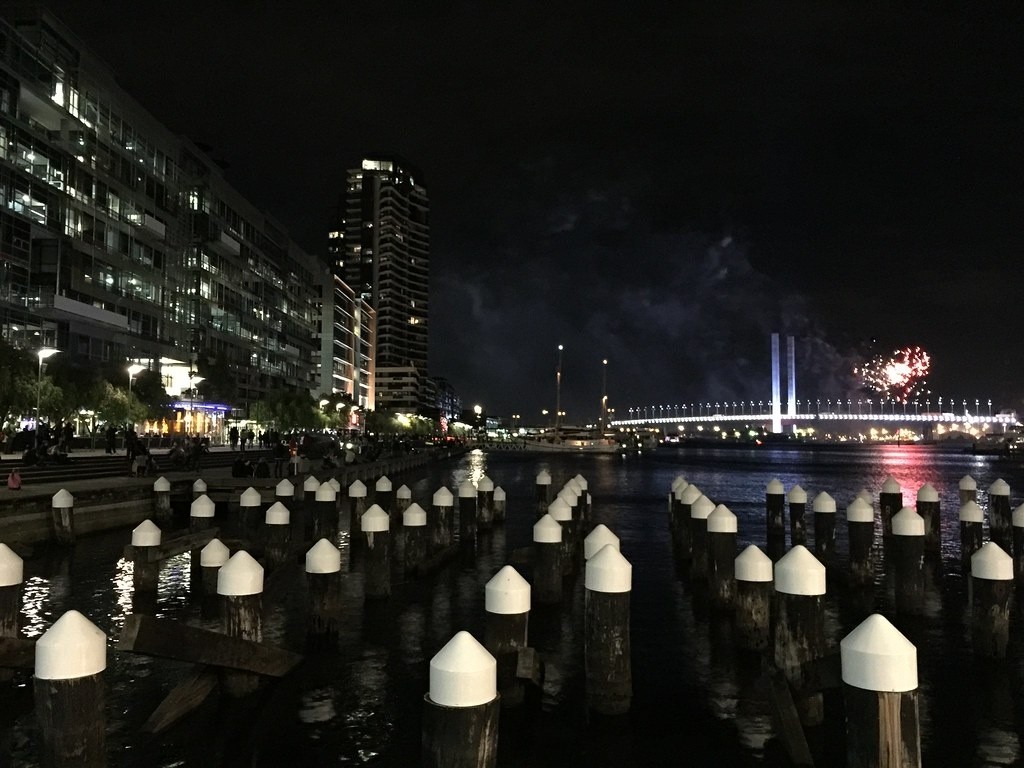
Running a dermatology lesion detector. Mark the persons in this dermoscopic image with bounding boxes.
[0,413,457,508]
